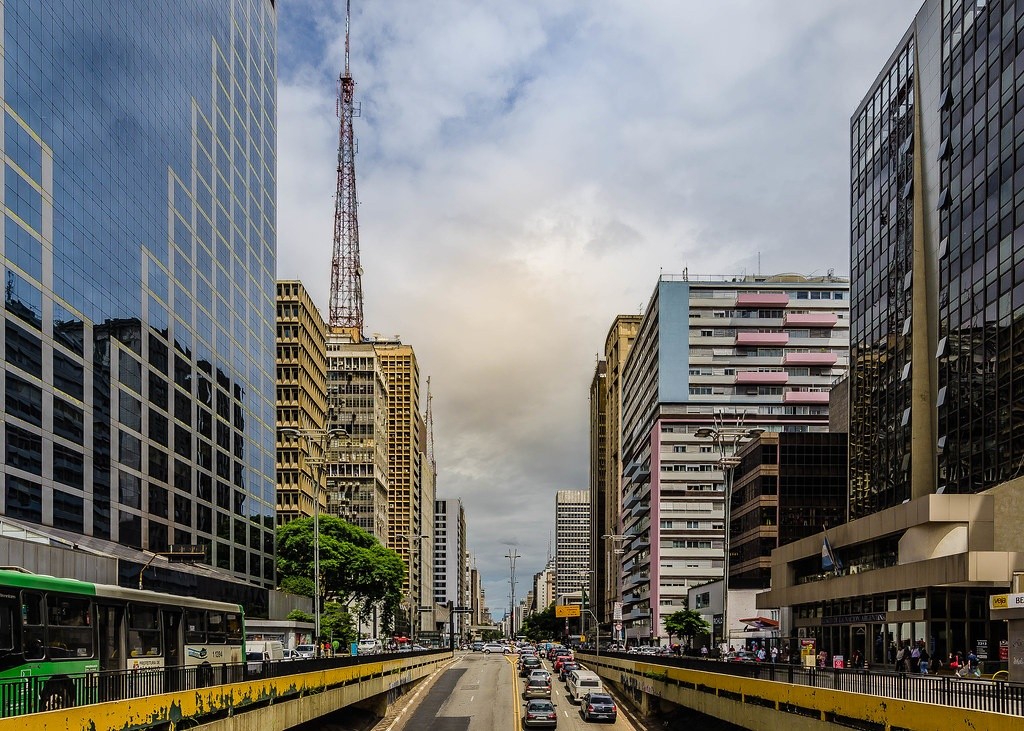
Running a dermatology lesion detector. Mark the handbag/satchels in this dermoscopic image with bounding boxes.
[975,657,981,666]
[950,655,958,669]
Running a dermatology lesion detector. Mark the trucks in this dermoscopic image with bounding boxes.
[564,669,603,704]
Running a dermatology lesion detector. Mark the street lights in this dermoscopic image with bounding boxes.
[395,533,429,651]
[600,533,636,651]
[572,570,595,651]
[279,427,350,659]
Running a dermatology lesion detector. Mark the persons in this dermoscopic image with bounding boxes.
[890,644,924,674]
[333,640,339,654]
[967,650,980,679]
[739,645,744,651]
[325,641,331,657]
[770,646,779,663]
[753,644,767,662]
[930,651,943,675]
[855,649,864,673]
[320,642,326,659]
[683,639,691,655]
[952,651,964,673]
[701,645,723,660]
[917,649,930,674]
[729,645,735,652]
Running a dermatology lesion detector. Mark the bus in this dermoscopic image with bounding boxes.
[0,562,246,721]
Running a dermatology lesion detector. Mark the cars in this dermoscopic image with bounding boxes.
[460,636,582,682]
[522,675,552,700]
[529,668,553,682]
[606,644,627,652]
[522,698,558,730]
[398,644,429,653]
[281,649,302,662]
[295,644,319,659]
[580,692,617,724]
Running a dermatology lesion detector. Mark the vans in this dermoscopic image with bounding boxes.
[357,638,383,656]
[246,640,284,673]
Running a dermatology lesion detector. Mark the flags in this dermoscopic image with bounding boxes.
[820,537,843,570]
